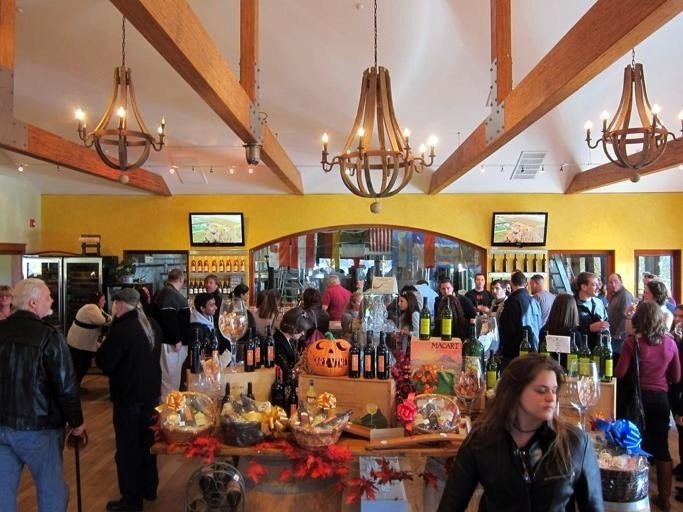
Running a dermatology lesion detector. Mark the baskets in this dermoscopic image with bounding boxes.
[220,420,274,447]
[401,394,461,447]
[600,465,649,503]
[289,407,349,449]
[157,391,216,444]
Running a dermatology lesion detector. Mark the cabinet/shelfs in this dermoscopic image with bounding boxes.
[189,250,251,297]
[22,255,118,338]
[486,248,549,293]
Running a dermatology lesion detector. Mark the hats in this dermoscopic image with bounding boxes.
[111,289,141,308]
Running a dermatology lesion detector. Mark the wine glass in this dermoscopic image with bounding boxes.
[366,403,378,424]
[474,315,500,382]
[219,298,248,376]
[361,294,395,331]
[629,298,639,318]
[194,355,224,400]
[568,359,600,433]
[453,356,480,417]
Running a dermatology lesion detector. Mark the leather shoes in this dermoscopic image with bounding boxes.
[143,490,154,502]
[675,493,682,500]
[105,499,143,512]
[674,463,682,472]
[677,474,683,481]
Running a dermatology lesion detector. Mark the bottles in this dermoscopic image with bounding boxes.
[222,470,242,512]
[287,370,299,418]
[462,319,485,392]
[198,279,202,294]
[363,330,375,379]
[211,256,217,272]
[241,255,245,272]
[503,254,508,272]
[513,253,518,271]
[306,379,318,404]
[567,331,579,377]
[297,399,310,429]
[254,332,261,369]
[492,254,497,272]
[219,256,224,272]
[425,396,440,431]
[486,349,497,391]
[198,467,221,509]
[538,329,551,359]
[533,254,539,272]
[541,254,546,272]
[222,277,227,294]
[592,333,601,377]
[519,328,532,357]
[523,254,529,272]
[243,328,255,372]
[220,382,232,412]
[203,280,207,294]
[579,334,592,376]
[189,394,207,428]
[203,256,208,272]
[207,329,218,362]
[197,256,202,273]
[246,381,255,402]
[348,331,361,378]
[218,279,222,294]
[440,297,453,341]
[600,335,614,383]
[193,279,198,295]
[191,256,196,272]
[419,297,431,340]
[271,366,286,409]
[226,256,231,272]
[264,324,274,367]
[233,256,239,272]
[227,278,230,294]
[189,278,193,295]
[376,330,389,380]
[444,396,458,429]
[483,354,487,383]
[320,408,354,427]
[164,262,168,274]
[190,328,202,374]
[180,399,195,426]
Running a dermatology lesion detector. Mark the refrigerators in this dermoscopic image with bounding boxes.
[20,255,119,375]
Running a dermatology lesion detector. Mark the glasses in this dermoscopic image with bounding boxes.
[401,290,407,297]
[302,312,312,320]
[1,291,12,296]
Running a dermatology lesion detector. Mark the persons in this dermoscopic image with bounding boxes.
[301,289,330,337]
[340,291,361,342]
[148,269,191,407]
[438,354,605,512]
[382,284,418,350]
[189,292,219,375]
[201,222,230,243]
[1,286,13,322]
[320,258,394,290]
[415,279,440,331]
[623,272,677,334]
[204,274,232,370]
[273,306,315,388]
[228,283,255,342]
[667,304,682,502]
[1,278,87,512]
[434,277,482,356]
[571,271,612,356]
[67,290,113,386]
[537,293,585,374]
[504,221,534,243]
[253,290,282,338]
[616,300,681,512]
[465,273,512,330]
[529,275,558,328]
[95,288,163,512]
[605,273,636,423]
[322,274,353,330]
[498,268,543,379]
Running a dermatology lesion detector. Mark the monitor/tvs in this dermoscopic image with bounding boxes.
[491,212,548,247]
[188,212,245,247]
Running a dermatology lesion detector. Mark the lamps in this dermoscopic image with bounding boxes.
[584,47,672,183]
[75,11,165,184]
[322,0,439,214]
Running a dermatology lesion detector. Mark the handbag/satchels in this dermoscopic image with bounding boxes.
[270,312,278,336]
[625,333,645,434]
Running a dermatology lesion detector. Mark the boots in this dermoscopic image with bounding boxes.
[651,461,674,512]
